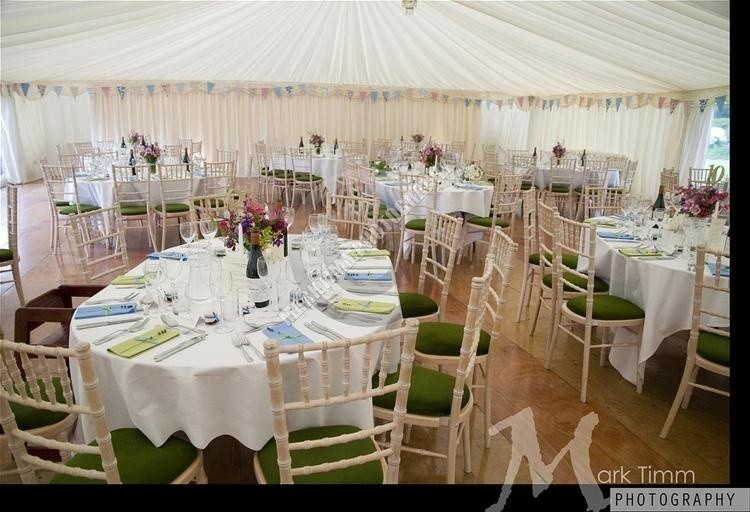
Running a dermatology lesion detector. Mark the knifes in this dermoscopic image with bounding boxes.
[303,317,351,347]
[74,315,144,330]
[148,334,207,362]
[347,287,401,297]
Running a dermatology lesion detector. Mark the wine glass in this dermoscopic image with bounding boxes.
[85,140,202,180]
[384,152,467,192]
[136,204,341,330]
[615,188,725,272]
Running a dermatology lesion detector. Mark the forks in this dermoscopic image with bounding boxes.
[86,289,138,307]
[228,334,266,364]
[326,306,384,326]
[91,317,151,345]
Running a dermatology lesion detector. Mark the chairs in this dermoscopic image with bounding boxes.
[0,125,731,485]
